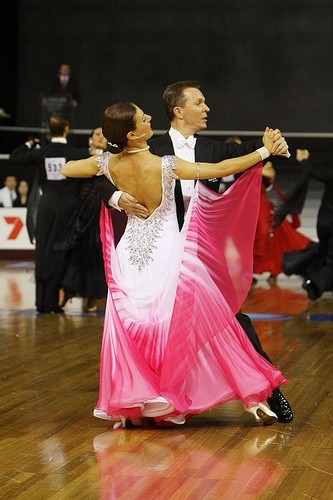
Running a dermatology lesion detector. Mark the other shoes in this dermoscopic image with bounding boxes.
[88,306,96,311]
[36,306,64,314]
[61,294,74,309]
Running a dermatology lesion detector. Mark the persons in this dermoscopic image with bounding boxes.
[61,102,280,428]
[49,63,80,107]
[218,138,333,300]
[0,114,107,315]
[101,80,293,423]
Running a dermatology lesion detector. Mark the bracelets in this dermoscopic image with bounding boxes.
[257,147,269,160]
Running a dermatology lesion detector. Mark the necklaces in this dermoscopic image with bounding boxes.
[124,146,149,153]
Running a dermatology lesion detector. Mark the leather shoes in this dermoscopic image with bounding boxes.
[267,391,294,423]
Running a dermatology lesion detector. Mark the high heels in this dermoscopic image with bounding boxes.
[244,402,278,425]
[120,414,144,429]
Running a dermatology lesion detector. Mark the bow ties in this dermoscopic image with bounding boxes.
[170,130,197,149]
